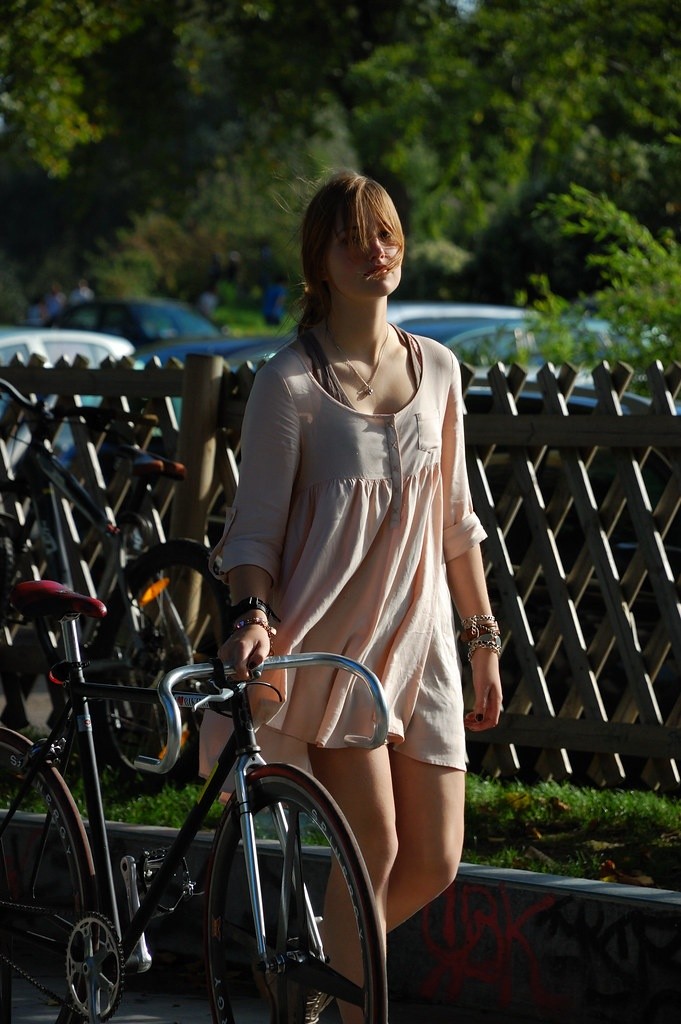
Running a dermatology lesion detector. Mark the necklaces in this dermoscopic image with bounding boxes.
[328,334,389,395]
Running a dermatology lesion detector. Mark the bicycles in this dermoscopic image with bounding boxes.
[0,377,389,1024]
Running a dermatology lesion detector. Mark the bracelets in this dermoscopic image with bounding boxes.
[226,619,277,639]
[461,613,501,660]
[225,597,282,624]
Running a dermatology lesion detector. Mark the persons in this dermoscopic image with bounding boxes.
[200,175,502,1024]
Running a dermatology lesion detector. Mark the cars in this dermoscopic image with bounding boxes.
[0,297,681,565]
